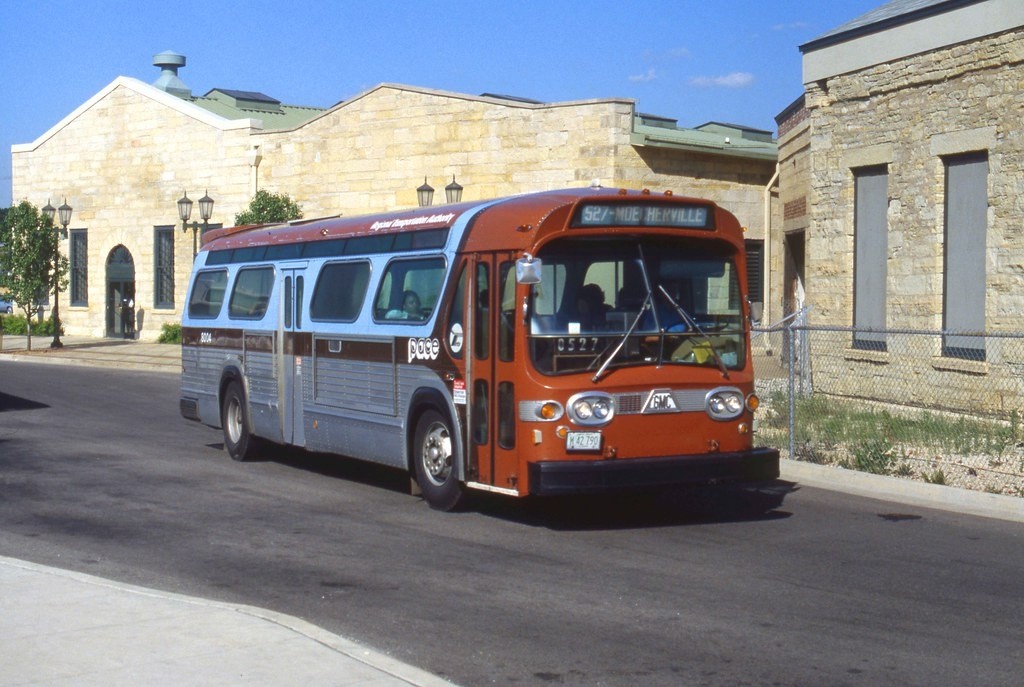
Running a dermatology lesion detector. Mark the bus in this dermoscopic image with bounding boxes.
[179,180,780,513]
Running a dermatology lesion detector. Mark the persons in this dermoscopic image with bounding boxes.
[643,278,694,345]
[118,291,134,339]
[479,289,512,331]
[574,284,614,331]
[385,290,427,323]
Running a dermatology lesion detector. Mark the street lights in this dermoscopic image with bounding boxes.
[42,196,73,348]
[178,187,215,265]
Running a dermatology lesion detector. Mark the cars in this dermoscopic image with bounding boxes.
[0,297,13,314]
